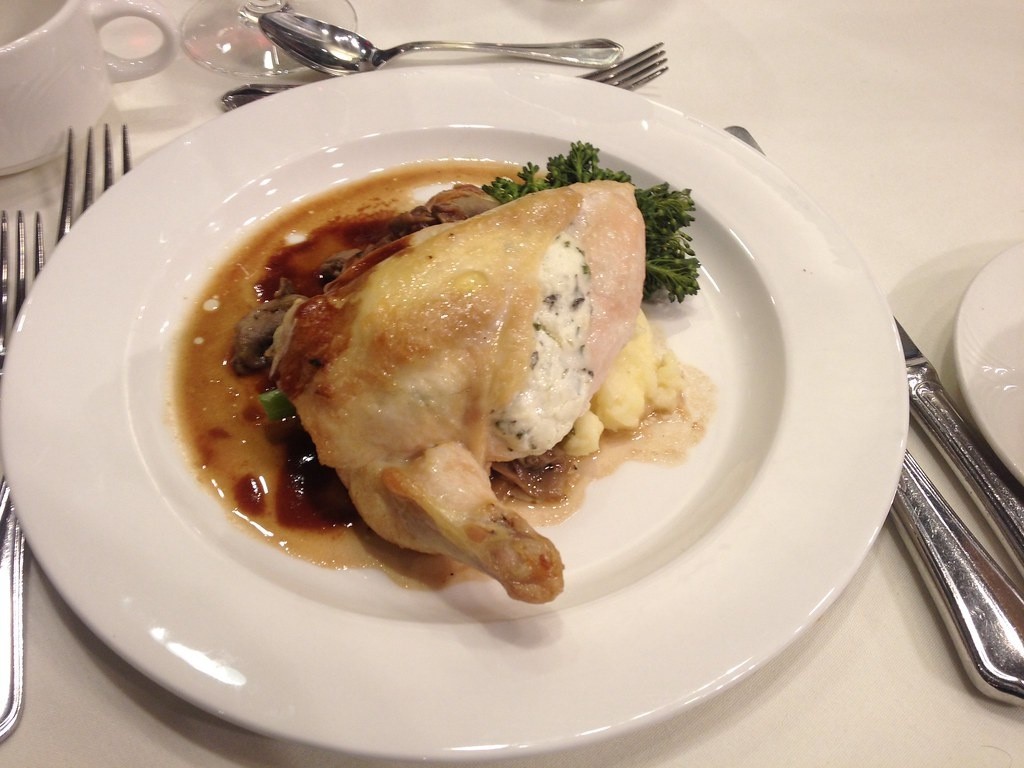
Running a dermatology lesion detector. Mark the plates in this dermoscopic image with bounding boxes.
[0,64,910,768]
[953,240,1024,484]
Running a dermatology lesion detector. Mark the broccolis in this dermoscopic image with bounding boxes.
[479,139,701,305]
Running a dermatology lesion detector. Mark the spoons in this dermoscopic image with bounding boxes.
[258,13,625,77]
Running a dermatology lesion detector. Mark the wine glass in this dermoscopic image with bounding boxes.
[180,0,358,76]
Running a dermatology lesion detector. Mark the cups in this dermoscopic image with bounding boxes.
[0,0,180,177]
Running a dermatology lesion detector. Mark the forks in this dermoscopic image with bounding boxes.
[0,209,45,738]
[56,123,130,242]
[221,42,669,111]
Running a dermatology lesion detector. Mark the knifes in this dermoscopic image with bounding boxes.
[726,125,1024,576]
